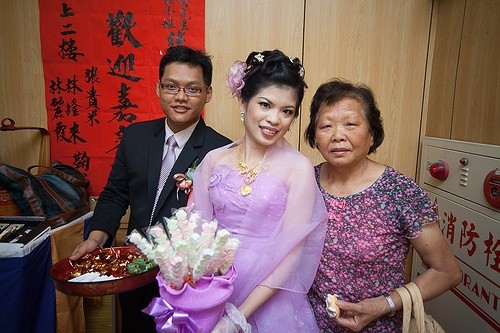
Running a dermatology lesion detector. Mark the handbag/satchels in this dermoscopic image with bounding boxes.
[393,282,446,333]
[0,160,91,229]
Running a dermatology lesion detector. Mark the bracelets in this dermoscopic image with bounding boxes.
[383,292,397,317]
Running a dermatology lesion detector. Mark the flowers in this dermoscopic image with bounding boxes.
[174,172,193,200]
[226,60,247,100]
[127,206,238,332]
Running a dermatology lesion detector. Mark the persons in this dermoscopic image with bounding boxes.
[188,50,327,333]
[70,45,237,333]
[303,79,463,333]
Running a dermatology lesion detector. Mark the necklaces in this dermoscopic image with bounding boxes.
[237,143,264,197]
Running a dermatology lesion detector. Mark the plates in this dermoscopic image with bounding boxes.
[49,246,160,296]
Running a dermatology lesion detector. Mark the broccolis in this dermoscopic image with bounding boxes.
[128,257,156,274]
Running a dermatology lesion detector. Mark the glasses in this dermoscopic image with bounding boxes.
[159,81,208,97]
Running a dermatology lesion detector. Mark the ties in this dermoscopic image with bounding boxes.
[150,134,177,226]
